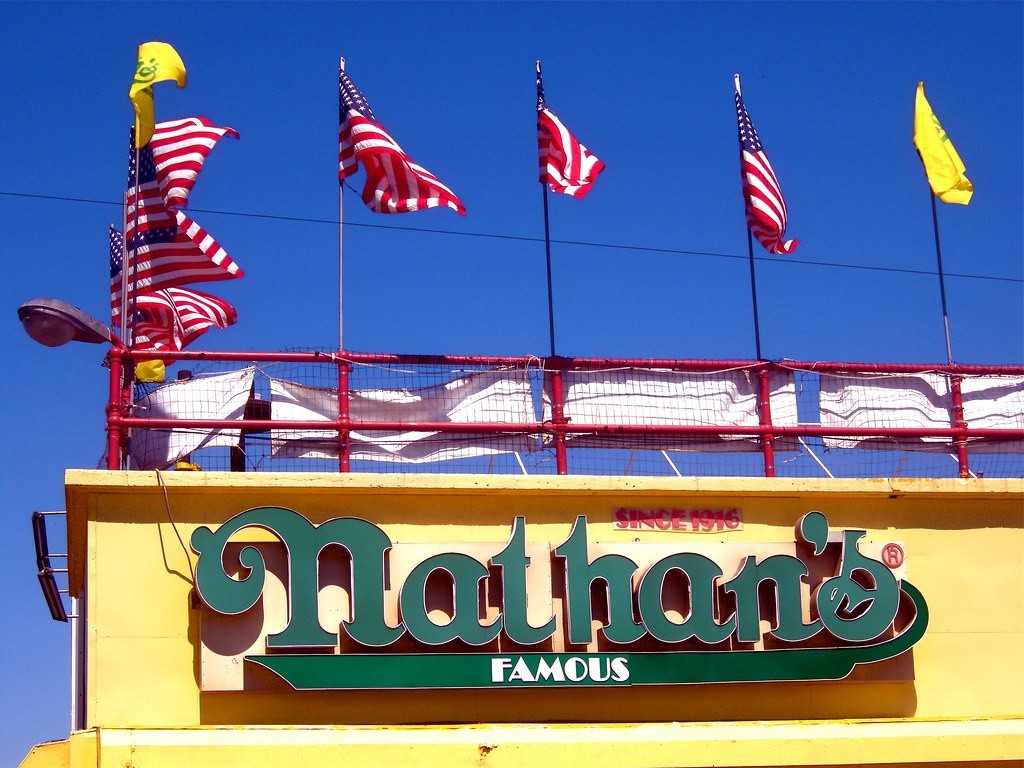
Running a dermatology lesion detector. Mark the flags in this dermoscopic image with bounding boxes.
[912,81,974,206]
[128,40,187,148]
[734,78,800,254]
[338,68,467,217]
[535,62,606,200]
[109,116,245,366]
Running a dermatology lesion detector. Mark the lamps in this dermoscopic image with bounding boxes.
[32,510,79,623]
[18,300,123,350]
